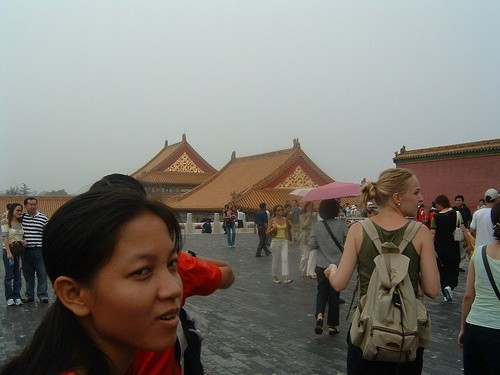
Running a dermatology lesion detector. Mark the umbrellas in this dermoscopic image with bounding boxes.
[302,181,361,200]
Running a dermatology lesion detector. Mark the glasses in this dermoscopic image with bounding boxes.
[275,209,283,211]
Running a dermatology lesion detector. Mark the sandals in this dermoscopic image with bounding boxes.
[315,315,323,334]
[328,327,340,335]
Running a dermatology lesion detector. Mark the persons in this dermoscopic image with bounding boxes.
[416,202,438,229]
[253,203,271,257]
[322,168,441,375]
[453,195,472,227]
[0,174,234,375]
[285,200,318,217]
[224,202,235,247]
[222,205,245,234]
[479,200,486,208]
[266,204,294,284]
[430,195,472,302]
[299,202,317,279]
[340,203,376,218]
[458,199,500,375]
[470,189,500,248]
[309,199,348,334]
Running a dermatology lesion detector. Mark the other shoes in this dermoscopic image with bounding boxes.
[444,286,453,301]
[266,252,271,255]
[256,255,264,257]
[310,275,316,278]
[273,280,280,283]
[284,280,293,284]
[301,272,306,276]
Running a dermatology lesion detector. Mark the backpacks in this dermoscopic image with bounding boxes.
[350,218,431,362]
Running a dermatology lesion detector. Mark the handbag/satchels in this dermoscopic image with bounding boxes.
[264,226,277,236]
[10,241,23,256]
[454,211,464,241]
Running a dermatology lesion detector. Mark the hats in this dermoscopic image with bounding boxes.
[485,188,500,200]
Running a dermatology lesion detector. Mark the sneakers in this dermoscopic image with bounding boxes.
[14,299,23,305]
[39,296,48,303]
[7,299,15,306]
[21,298,34,303]
